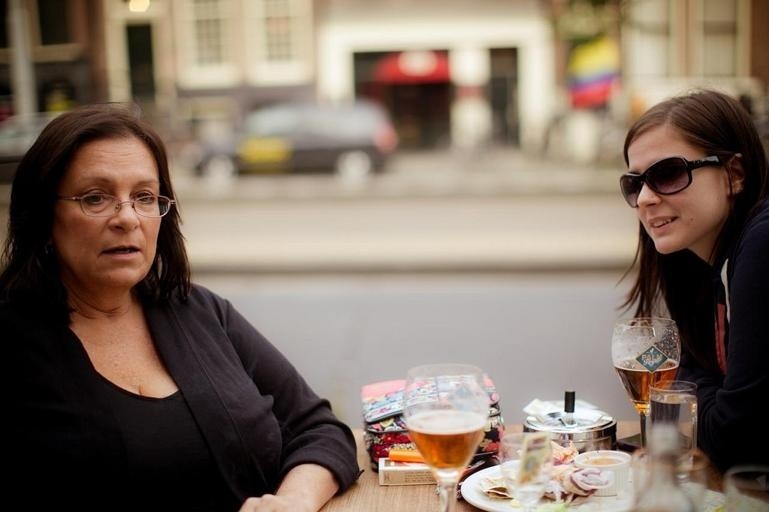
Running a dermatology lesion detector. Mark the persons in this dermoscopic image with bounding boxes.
[611,88,769,481]
[0,105,361,510]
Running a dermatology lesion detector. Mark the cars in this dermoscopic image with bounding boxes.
[0,111,65,184]
[194,98,399,183]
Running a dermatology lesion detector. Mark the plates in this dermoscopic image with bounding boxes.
[574,449,631,469]
[460,459,630,511]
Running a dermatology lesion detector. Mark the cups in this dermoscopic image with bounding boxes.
[648,379,699,450]
[631,450,711,511]
[499,433,555,512]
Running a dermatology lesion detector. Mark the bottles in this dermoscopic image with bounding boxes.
[632,421,696,512]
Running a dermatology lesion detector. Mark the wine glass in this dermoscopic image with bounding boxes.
[611,316,682,448]
[401,362,491,511]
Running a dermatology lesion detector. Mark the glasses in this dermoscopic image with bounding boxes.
[58,193,179,218]
[619,155,719,209]
